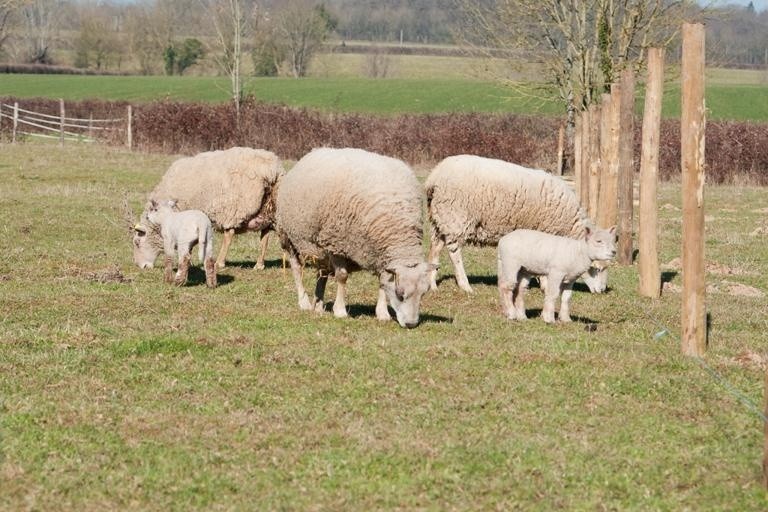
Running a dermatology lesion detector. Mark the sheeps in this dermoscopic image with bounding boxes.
[146,198,217,288]
[494,223,620,324]
[271,144,443,330]
[423,153,610,297]
[127,144,289,271]
